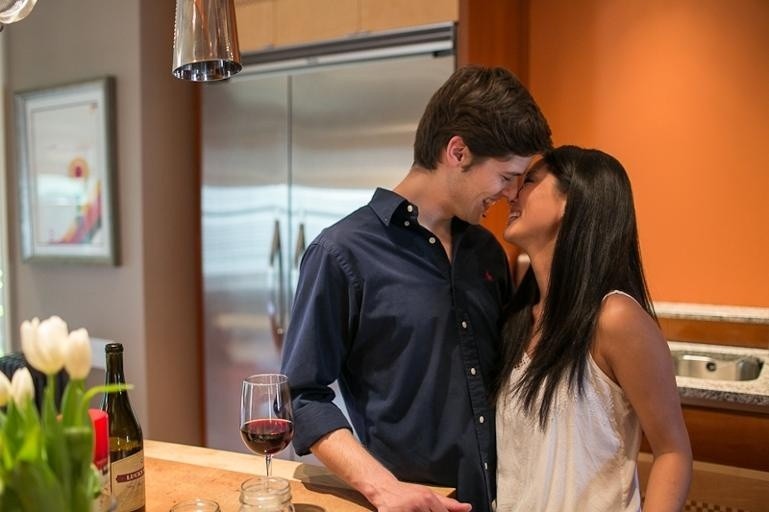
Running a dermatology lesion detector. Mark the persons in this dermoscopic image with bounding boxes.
[494,144,692,512]
[275,65,555,512]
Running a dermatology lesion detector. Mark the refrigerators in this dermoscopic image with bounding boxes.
[196,18,457,467]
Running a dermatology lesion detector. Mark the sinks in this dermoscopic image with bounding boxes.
[669,348,765,380]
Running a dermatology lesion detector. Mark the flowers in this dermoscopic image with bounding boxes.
[0,316,134,512]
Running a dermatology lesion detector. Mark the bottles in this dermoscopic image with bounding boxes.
[235,474,296,512]
[99,342,146,512]
[169,497,222,512]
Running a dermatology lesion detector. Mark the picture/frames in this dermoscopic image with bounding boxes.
[9,77,125,269]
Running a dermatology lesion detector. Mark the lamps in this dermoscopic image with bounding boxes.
[170,1,242,87]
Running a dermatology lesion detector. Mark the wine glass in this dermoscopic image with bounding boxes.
[238,373,296,477]
